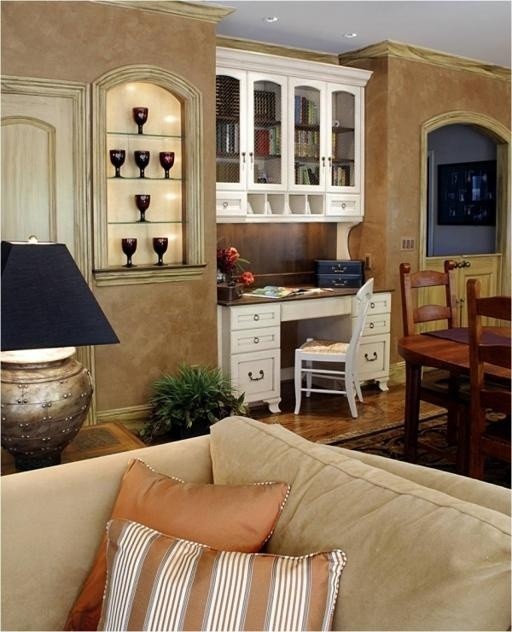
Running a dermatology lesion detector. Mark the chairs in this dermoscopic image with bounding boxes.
[399,259,462,444]
[461,276,511,481]
[292,276,376,418]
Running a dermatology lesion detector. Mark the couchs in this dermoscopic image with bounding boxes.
[2,411,510,630]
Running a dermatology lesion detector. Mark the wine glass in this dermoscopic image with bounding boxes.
[108,149,126,178]
[133,106,148,134]
[121,237,138,269]
[152,237,170,266]
[159,152,175,178]
[134,194,151,222]
[134,150,150,178]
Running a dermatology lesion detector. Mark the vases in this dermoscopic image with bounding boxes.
[218,281,243,301]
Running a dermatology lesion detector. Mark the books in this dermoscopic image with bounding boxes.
[216,76,351,188]
[243,285,311,299]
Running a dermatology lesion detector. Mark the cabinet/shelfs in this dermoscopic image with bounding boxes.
[88,63,207,289]
[216,190,368,225]
[287,56,373,190]
[216,49,287,190]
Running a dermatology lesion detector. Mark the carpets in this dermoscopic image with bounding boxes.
[315,402,510,490]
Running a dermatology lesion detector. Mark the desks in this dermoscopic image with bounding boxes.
[398,324,511,481]
[2,418,149,479]
[216,281,397,415]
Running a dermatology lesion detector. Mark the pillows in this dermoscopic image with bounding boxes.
[99,516,348,630]
[61,454,292,631]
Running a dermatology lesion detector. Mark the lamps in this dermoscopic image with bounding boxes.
[217,246,256,287]
[2,234,123,470]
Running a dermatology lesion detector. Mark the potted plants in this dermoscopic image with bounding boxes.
[136,362,248,444]
